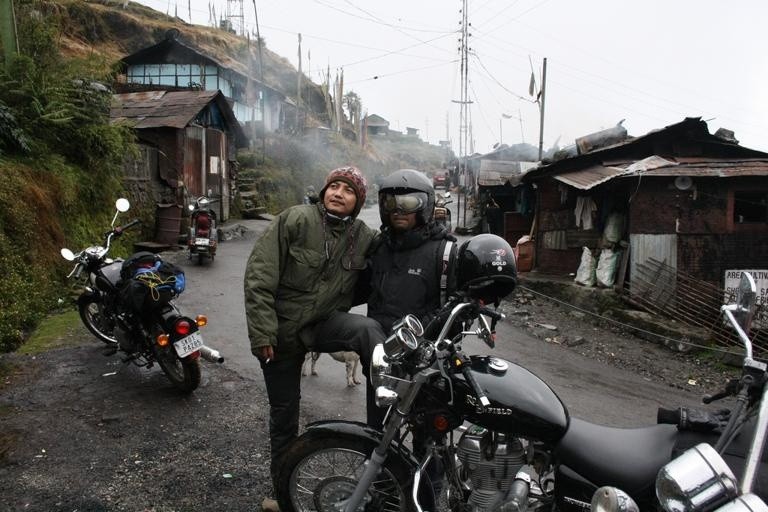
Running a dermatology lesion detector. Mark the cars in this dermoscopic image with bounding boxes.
[433,169,449,189]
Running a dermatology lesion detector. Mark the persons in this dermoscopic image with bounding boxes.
[241,166,404,512]
[444,172,451,192]
[351,169,462,512]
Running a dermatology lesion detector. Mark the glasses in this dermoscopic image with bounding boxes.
[380,194,425,211]
[323,212,353,226]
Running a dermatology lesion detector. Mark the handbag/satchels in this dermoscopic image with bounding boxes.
[114,251,186,307]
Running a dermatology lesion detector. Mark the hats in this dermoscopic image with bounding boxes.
[317,167,367,218]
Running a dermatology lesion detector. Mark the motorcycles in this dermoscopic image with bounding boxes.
[187,188,222,266]
[61,197,223,394]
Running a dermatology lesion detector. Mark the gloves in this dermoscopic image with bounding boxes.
[656,406,741,437]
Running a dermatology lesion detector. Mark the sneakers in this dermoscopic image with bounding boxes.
[262,498,279,511]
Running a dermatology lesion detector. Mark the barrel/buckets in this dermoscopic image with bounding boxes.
[155,207,181,249]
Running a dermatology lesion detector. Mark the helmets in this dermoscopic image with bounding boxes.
[378,169,435,228]
[456,233,517,306]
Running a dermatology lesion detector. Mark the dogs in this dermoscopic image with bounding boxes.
[302,351,361,387]
